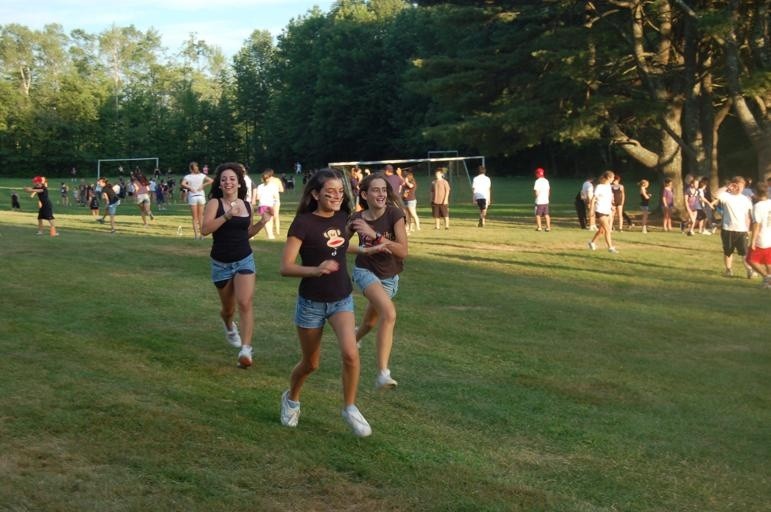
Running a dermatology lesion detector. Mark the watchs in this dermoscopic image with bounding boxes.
[371,232,382,243]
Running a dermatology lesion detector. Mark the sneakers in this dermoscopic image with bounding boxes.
[341,404,372,438]
[281,390,301,426]
[97,217,115,233]
[354,327,362,348]
[376,368,397,394]
[478,217,484,226]
[680,223,717,235]
[221,319,243,347]
[536,228,551,232]
[722,265,771,289]
[148,212,153,221]
[609,247,618,253]
[35,231,60,236]
[588,241,596,250]
[238,346,254,369]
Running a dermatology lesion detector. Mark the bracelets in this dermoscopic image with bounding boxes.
[223,213,229,221]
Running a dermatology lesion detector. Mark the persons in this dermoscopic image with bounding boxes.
[430,168,451,230]
[471,166,491,227]
[59,161,214,241]
[532,168,551,231]
[23,176,56,237]
[10,189,21,211]
[255,174,277,240]
[349,164,420,237]
[201,163,274,369]
[239,164,256,232]
[264,168,285,236]
[276,160,319,190]
[344,174,409,390]
[572,169,771,287]
[280,167,373,439]
